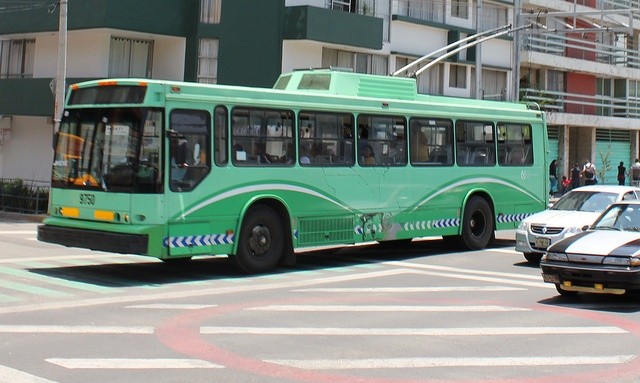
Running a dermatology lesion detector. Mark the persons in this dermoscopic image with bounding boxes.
[363,144,375,164]
[570,162,583,188]
[157,137,196,192]
[411,120,429,161]
[561,176,571,194]
[617,161,628,184]
[252,142,273,163]
[286,139,310,165]
[627,157,640,186]
[580,160,596,185]
[549,158,559,196]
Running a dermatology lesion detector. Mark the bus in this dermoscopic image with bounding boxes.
[38,66,551,275]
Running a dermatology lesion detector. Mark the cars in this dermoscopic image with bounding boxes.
[515,184,640,261]
[540,200,640,301]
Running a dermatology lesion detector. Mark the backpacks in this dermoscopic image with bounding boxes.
[585,163,594,178]
